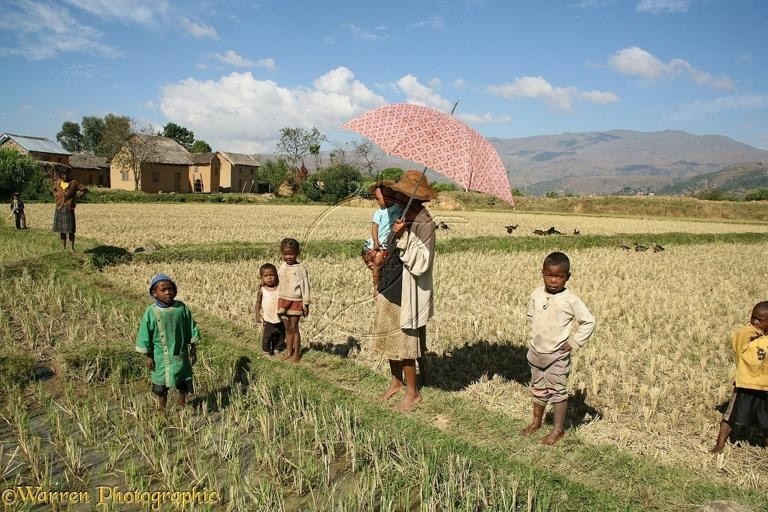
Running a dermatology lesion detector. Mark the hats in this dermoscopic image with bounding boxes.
[14,191,21,196]
[390,169,438,202]
[149,273,177,296]
[367,178,397,195]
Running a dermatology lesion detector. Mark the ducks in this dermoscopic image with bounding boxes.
[504,224,519,234]
[433,220,450,230]
[634,242,649,252]
[622,245,630,252]
[573,229,579,235]
[652,241,666,253]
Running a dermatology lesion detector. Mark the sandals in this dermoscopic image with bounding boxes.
[261,349,301,363]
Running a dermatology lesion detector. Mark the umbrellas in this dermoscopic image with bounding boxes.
[336,95,515,245]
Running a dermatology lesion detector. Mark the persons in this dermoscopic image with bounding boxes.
[518,250,597,444]
[134,274,203,411]
[256,237,310,364]
[52,167,89,254]
[708,301,768,455]
[10,192,30,229]
[364,169,440,413]
[360,237,430,385]
[366,176,428,298]
[254,263,286,359]
